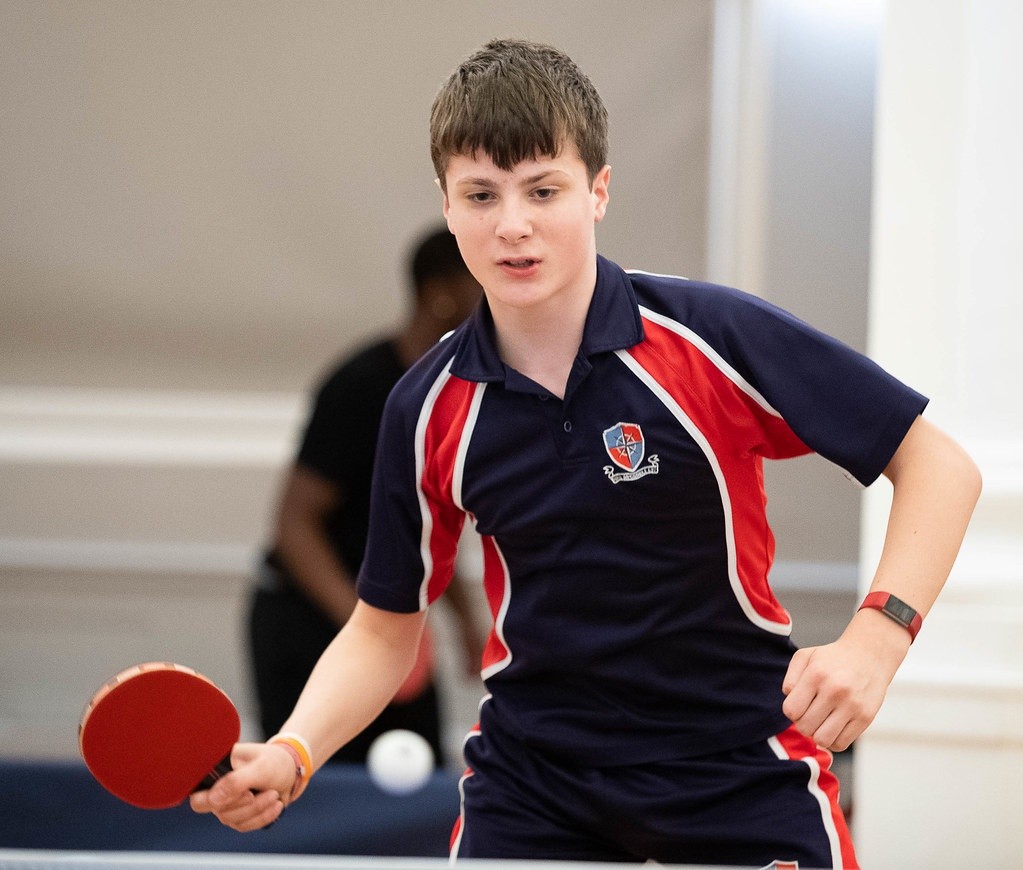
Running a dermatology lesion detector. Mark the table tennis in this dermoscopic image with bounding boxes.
[367,729,436,798]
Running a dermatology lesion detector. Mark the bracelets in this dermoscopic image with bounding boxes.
[266,733,312,802]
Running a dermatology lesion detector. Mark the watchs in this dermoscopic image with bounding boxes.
[857,591,923,644]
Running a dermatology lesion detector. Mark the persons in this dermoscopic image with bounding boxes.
[246,226,484,766]
[188,39,984,870]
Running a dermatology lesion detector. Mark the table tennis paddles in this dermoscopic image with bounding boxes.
[79,662,283,829]
